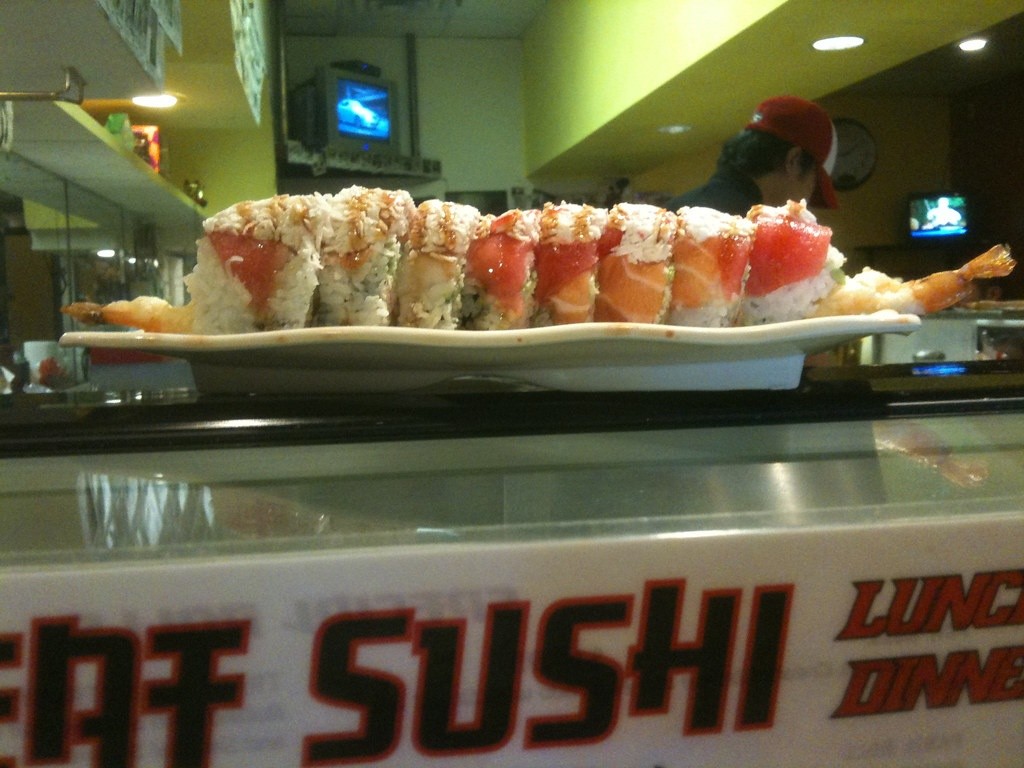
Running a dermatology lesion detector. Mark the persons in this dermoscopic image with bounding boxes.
[660,96,839,218]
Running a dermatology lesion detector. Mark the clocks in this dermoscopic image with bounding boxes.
[832,117,882,190]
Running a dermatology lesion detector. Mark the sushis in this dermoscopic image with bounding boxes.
[193,183,851,334]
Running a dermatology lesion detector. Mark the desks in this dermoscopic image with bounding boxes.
[853,239,978,278]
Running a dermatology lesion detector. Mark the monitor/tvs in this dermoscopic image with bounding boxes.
[906,192,974,246]
[289,66,400,156]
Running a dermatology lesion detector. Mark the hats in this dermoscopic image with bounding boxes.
[744,96,839,209]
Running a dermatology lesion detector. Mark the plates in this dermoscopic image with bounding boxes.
[58,308,922,395]
